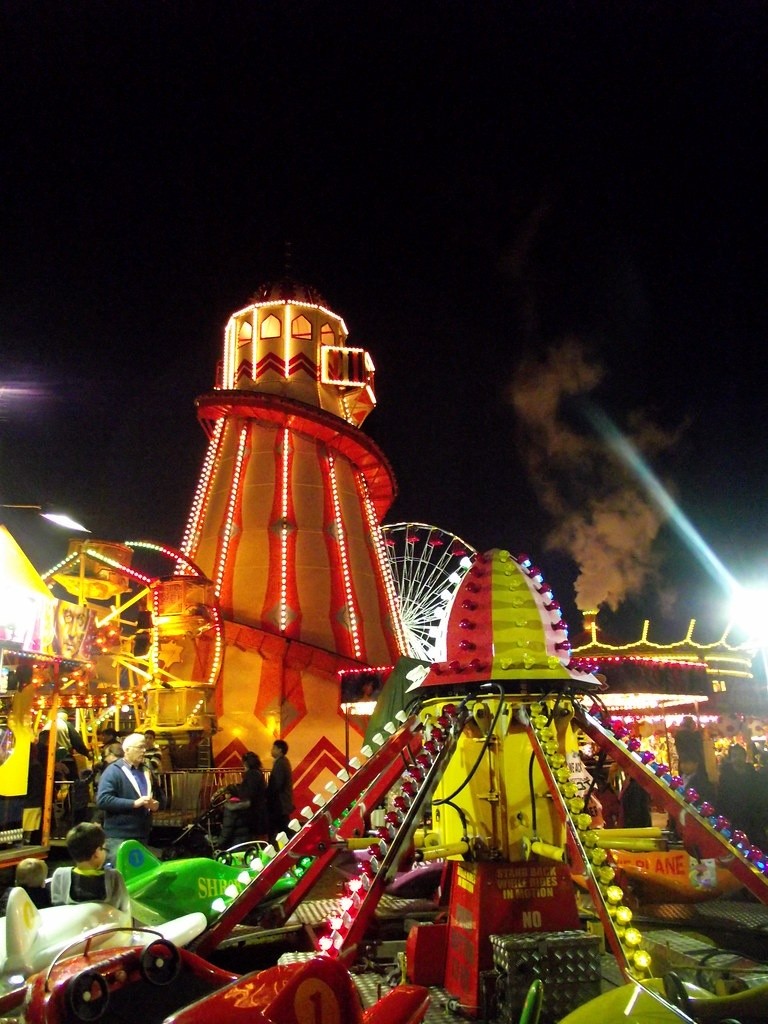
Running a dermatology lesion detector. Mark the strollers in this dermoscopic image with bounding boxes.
[160,783,227,860]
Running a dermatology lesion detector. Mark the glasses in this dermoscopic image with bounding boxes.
[102,848,110,855]
[129,745,149,751]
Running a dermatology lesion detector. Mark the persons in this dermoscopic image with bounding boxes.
[206,752,271,848]
[621,776,652,828]
[755,750,768,843]
[143,730,163,810]
[217,784,257,846]
[678,752,712,809]
[675,716,704,752]
[97,733,166,848]
[0,701,123,808]
[718,744,757,835]
[267,740,292,846]
[51,823,132,929]
[0,857,52,916]
[592,775,622,830]
